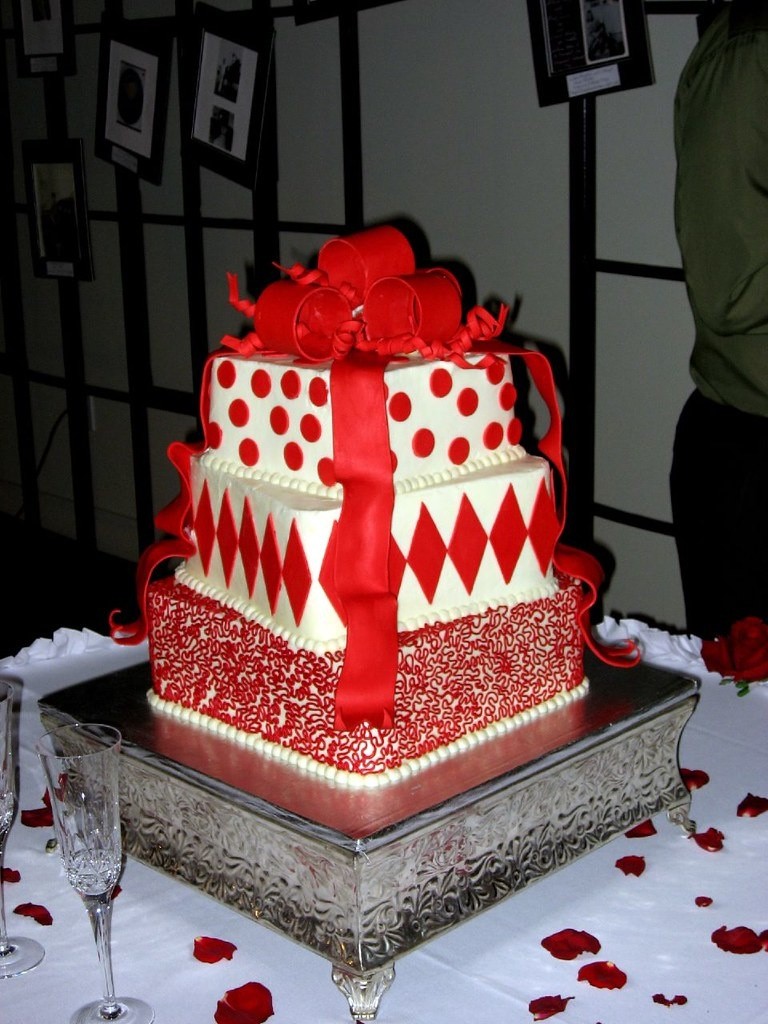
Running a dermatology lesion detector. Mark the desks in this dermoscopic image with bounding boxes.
[0,632,768,1024]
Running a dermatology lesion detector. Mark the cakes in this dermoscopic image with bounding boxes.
[105,231,642,845]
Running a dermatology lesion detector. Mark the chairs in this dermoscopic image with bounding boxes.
[179,2,276,192]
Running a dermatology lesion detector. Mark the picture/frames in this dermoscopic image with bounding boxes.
[22,138,96,283]
[12,0,78,78]
[291,0,406,27]
[526,0,655,108]
[93,10,173,187]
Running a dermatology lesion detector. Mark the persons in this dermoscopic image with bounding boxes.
[669,0,767,645]
[216,53,242,97]
[584,10,603,47]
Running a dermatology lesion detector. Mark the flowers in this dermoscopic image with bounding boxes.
[700,617,768,696]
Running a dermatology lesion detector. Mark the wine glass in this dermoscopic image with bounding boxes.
[0,678,46,979]
[37,722,154,1024]
